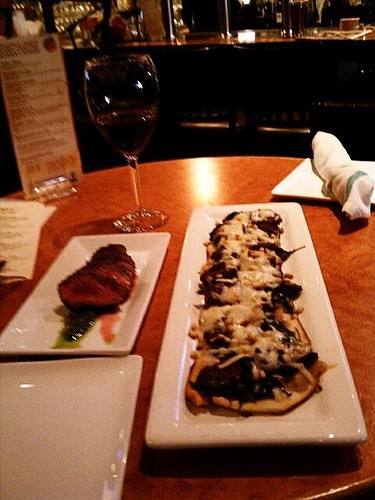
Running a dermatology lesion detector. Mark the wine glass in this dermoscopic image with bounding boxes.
[85,53,170,232]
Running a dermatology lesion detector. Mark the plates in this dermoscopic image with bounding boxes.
[144,202,368,450]
[0,354,144,500]
[270,158,375,204]
[0,233,172,355]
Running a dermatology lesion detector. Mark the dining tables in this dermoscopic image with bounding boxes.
[0,156,375,500]
